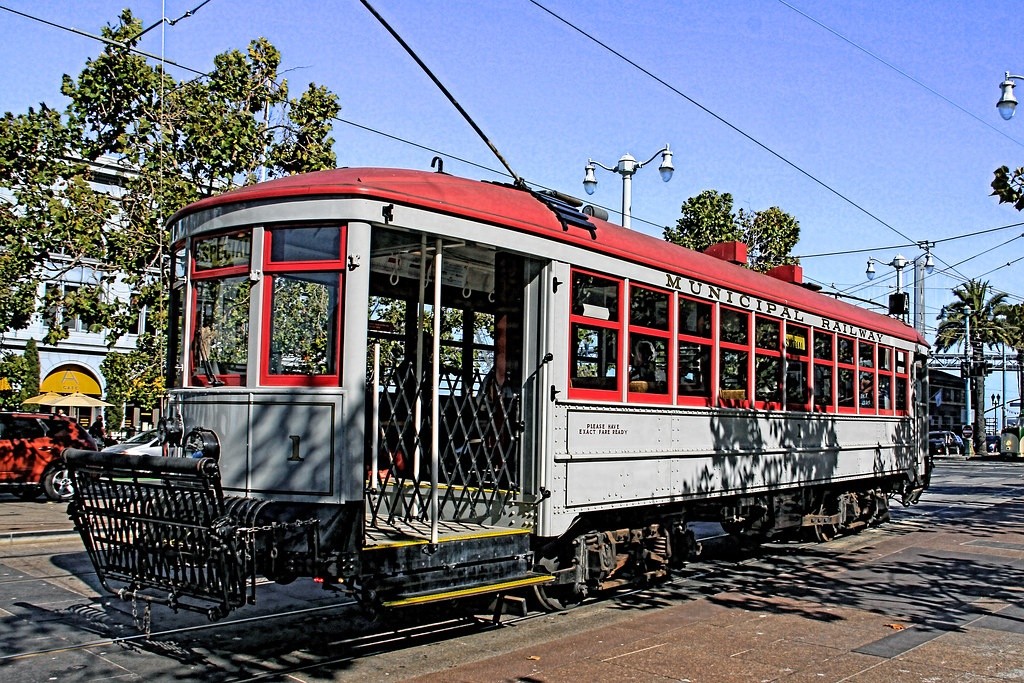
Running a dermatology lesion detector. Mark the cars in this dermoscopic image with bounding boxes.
[101,426,163,455]
[999,409,1024,461]
[973,435,1007,461]
[927,430,966,456]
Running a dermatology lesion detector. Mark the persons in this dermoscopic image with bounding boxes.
[89,414,105,448]
[58,410,67,418]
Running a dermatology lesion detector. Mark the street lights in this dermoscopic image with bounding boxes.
[990,393,1001,437]
[581,142,675,230]
[865,251,935,320]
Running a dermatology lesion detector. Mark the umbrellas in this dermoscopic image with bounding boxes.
[45,390,116,423]
[20,390,64,413]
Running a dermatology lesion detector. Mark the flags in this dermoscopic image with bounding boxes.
[935,391,943,407]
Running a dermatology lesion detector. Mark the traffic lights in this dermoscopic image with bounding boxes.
[984,361,993,377]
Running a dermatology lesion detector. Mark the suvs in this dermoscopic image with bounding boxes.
[0,411,99,502]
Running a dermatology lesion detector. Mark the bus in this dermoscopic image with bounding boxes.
[59,166,932,627]
